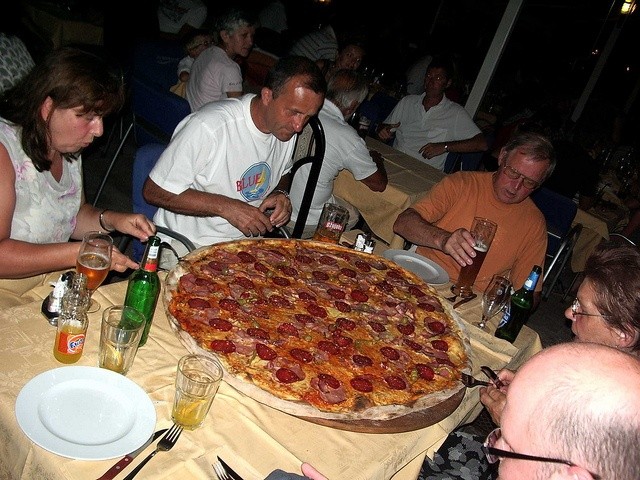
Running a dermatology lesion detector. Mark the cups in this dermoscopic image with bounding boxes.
[99,305,147,376]
[450,217,497,298]
[312,203,349,244]
[172,354,224,430]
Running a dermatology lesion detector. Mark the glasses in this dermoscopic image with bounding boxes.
[481,428,601,480]
[572,297,612,318]
[504,158,539,189]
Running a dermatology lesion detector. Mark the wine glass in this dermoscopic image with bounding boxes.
[76,230,114,313]
[471,274,513,334]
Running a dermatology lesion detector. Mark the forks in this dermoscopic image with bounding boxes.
[123,423,183,479]
[212,461,233,480]
[461,373,494,388]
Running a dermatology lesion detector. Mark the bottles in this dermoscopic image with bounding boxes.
[53,273,90,364]
[116,236,161,347]
[495,265,542,344]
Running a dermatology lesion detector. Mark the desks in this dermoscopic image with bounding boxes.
[170,83,449,251]
[561,197,622,273]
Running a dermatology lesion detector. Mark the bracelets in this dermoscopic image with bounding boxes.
[270,189,291,201]
[444,139,450,155]
[98,209,115,235]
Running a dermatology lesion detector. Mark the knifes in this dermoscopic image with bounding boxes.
[453,294,477,309]
[96,429,167,480]
[217,456,244,480]
[480,365,504,388]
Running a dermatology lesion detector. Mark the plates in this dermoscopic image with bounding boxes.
[382,248,449,286]
[15,365,157,461]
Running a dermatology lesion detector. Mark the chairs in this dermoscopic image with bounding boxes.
[530,184,582,301]
[89,80,191,208]
[99,146,196,284]
[561,223,639,305]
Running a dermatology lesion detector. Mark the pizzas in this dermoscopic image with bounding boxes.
[167,238,470,414]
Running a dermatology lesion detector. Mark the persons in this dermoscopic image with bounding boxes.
[393,133,555,313]
[290,16,352,60]
[263,344,639,479]
[143,56,324,261]
[149,1,208,46]
[252,2,294,53]
[185,16,254,113]
[0,42,154,282]
[285,69,389,242]
[315,43,370,86]
[176,27,215,83]
[480,246,639,430]
[375,61,487,174]
